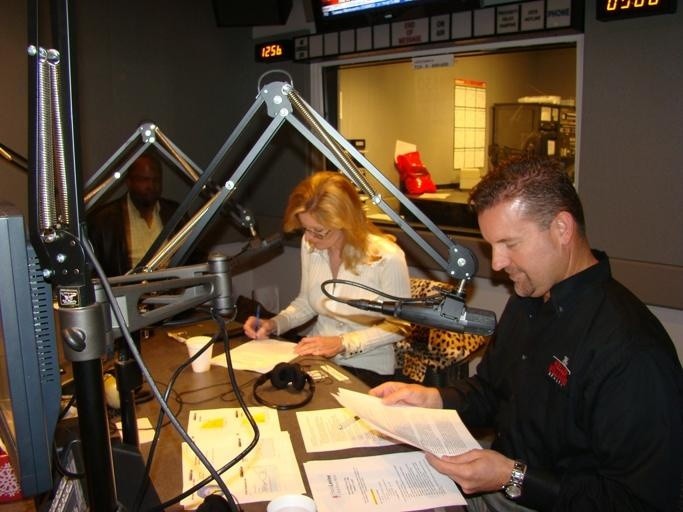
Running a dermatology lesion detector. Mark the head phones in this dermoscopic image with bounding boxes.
[252,361,316,409]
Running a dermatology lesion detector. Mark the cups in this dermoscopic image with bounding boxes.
[184,335,214,373]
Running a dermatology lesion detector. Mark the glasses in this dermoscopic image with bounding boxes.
[302,225,331,239]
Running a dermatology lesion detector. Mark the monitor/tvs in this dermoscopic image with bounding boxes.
[1,198,62,498]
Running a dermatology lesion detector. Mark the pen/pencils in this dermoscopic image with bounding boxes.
[337,416,360,430]
[255,304,260,342]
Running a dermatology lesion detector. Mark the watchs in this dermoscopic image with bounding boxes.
[500,460,528,501]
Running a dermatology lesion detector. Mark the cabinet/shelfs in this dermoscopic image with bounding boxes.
[492,103,577,183]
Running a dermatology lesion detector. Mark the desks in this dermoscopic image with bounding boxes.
[2,306,466,512]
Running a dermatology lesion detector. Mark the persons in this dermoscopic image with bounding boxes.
[360,152,682,512]
[241,170,412,392]
[86,144,216,322]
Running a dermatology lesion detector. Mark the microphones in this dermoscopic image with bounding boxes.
[227,230,286,276]
[377,296,498,340]
